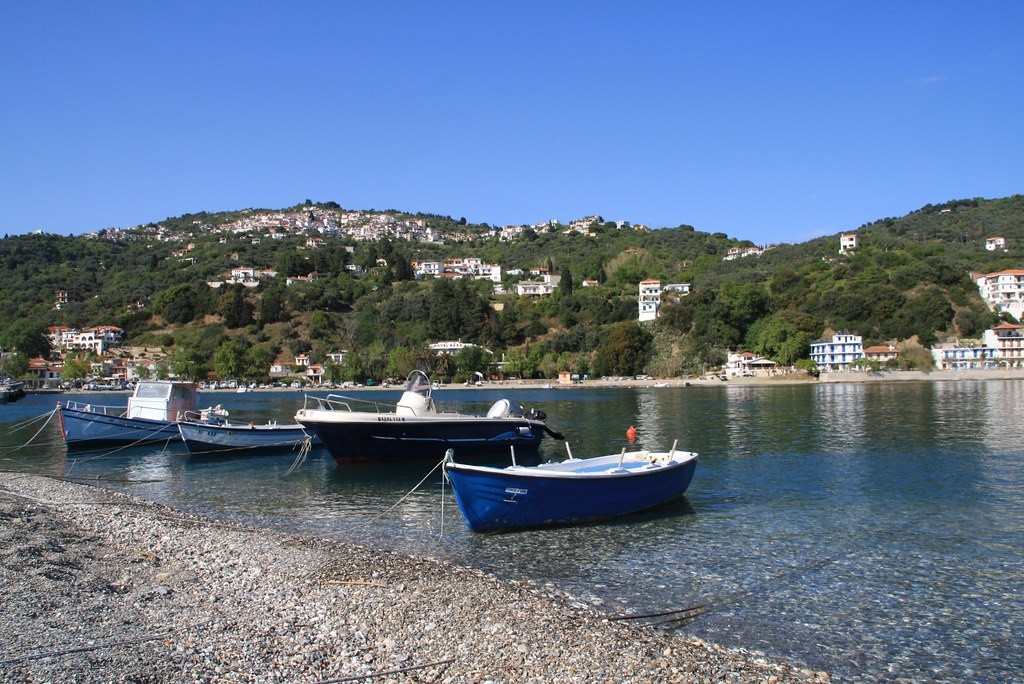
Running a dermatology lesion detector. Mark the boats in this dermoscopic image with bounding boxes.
[0,378,24,401]
[57,379,200,445]
[176,410,324,458]
[443,439,700,532]
[293,370,548,468]
